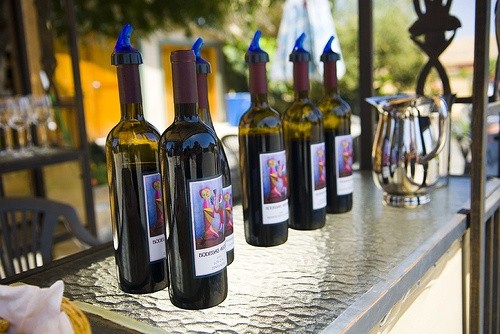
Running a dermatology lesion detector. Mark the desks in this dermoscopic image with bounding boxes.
[0,171,500,334]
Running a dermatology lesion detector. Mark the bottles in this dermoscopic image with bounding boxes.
[191,37,234,266]
[105,24,168,295]
[238,30,289,247]
[281,32,327,231]
[158,49,228,311]
[316,36,353,214]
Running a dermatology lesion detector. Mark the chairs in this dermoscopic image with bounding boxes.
[0,196,100,277]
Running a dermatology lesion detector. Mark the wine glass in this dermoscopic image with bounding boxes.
[0,93,38,158]
[26,95,56,153]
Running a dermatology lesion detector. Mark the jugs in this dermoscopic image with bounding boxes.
[365,95,449,207]
